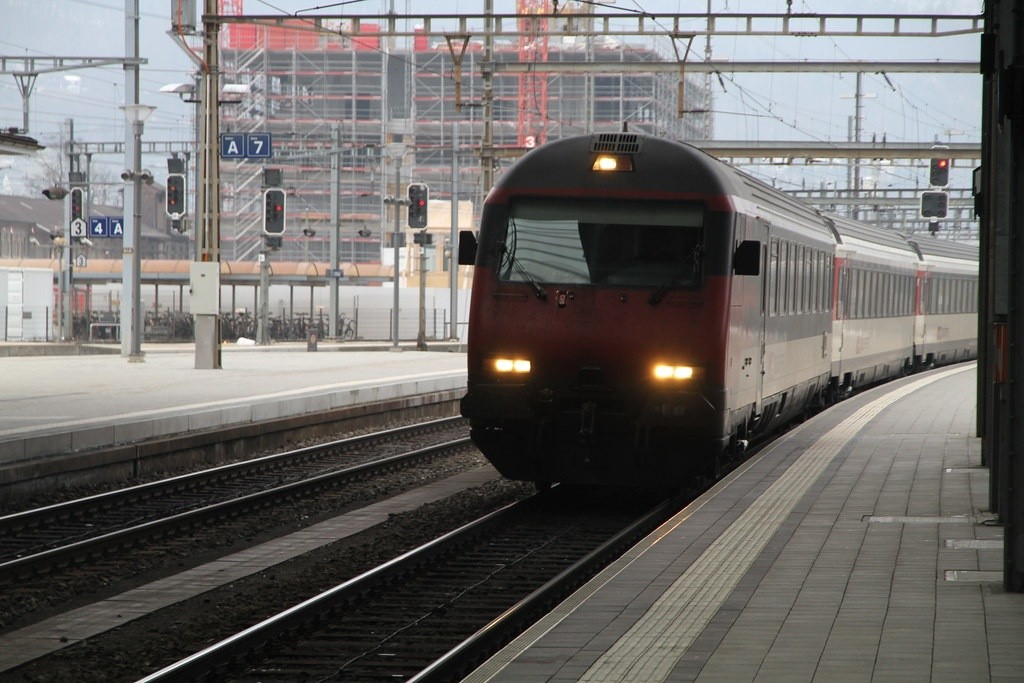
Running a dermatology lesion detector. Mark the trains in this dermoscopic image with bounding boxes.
[458,121,979,496]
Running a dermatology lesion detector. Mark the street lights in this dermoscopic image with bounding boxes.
[121,103,157,362]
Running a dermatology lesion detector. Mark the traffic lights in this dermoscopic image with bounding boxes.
[70,187,83,218]
[165,176,186,216]
[408,184,428,229]
[931,158,947,186]
[265,190,285,234]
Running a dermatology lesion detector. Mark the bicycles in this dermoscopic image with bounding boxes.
[144,307,195,341]
[222,311,257,339]
[267,310,356,341]
[73,309,120,343]
